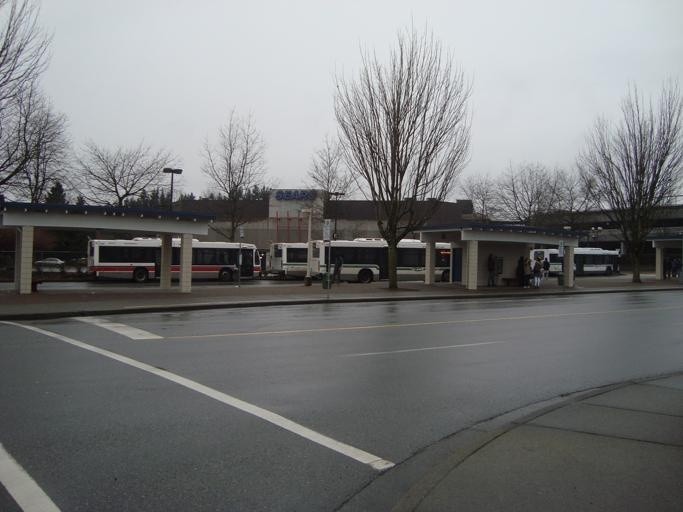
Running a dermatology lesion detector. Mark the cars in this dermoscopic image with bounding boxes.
[34,258,65,267]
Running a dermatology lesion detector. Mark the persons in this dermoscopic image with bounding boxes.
[332,253,344,283]
[517,255,542,288]
[543,258,550,280]
[487,253,496,287]
[663,256,681,279]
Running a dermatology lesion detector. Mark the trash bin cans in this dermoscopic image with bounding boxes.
[558,273,564,285]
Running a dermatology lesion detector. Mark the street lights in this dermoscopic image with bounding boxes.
[329,192,345,240]
[164,167,183,209]
[592,226,603,247]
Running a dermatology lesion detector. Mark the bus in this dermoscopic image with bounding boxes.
[270,238,451,283]
[88,238,262,282]
[530,248,620,275]
